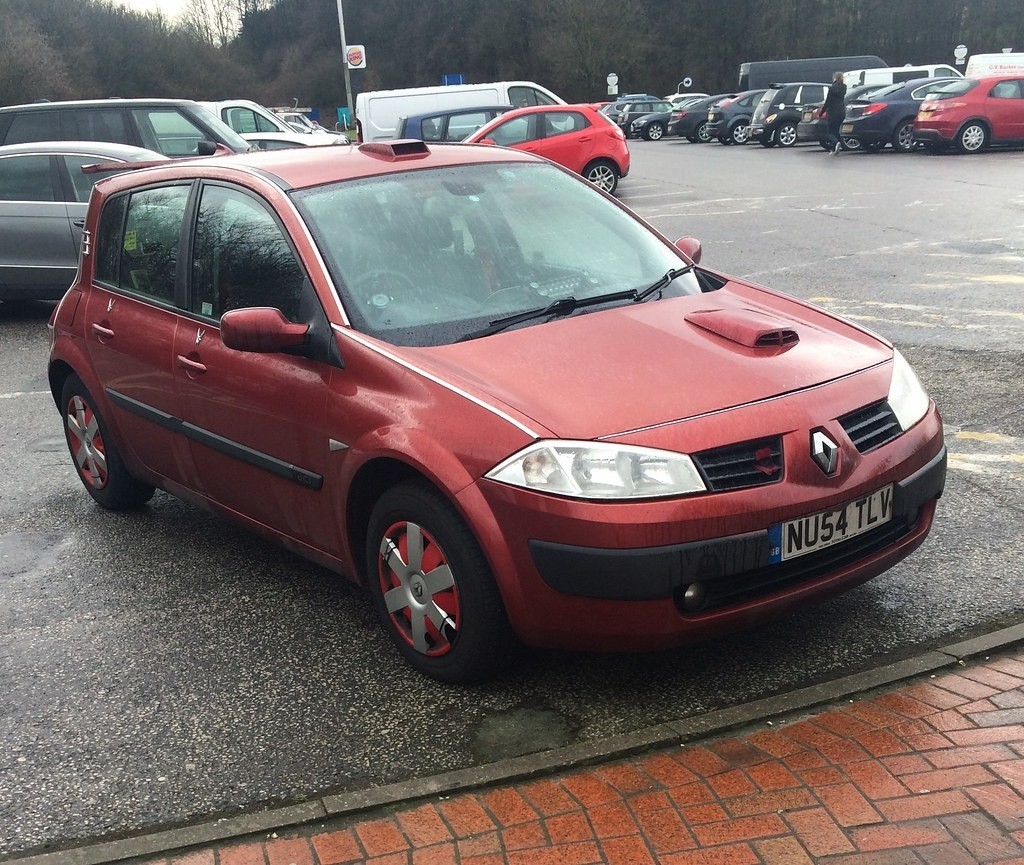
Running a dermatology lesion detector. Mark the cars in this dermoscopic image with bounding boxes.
[839,76,965,153]
[912,74,1024,154]
[746,83,832,148]
[48,139,948,683]
[601,56,769,145]
[0,82,630,301]
[797,84,893,151]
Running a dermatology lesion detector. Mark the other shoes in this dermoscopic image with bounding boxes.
[835,141,842,155]
[829,150,835,156]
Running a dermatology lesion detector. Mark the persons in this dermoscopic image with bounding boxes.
[819,71,847,156]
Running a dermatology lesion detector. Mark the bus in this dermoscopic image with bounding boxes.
[736,56,889,94]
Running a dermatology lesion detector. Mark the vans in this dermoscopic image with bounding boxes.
[843,64,965,93]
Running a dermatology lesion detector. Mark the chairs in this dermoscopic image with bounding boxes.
[993,86,1002,98]
[387,198,464,296]
